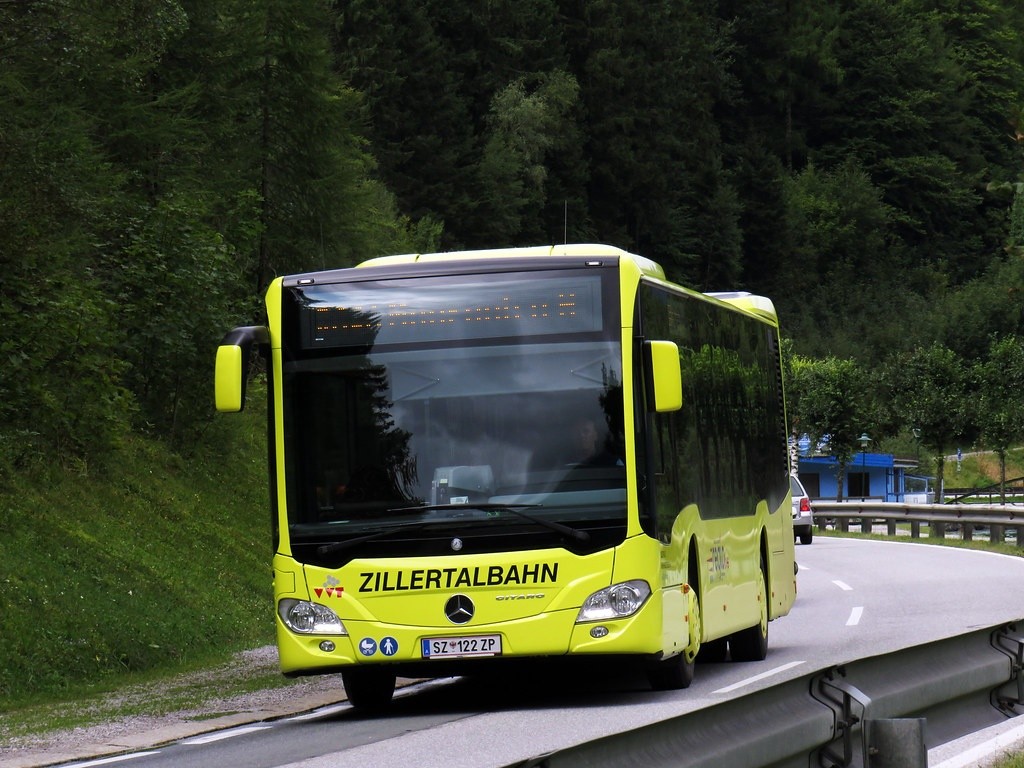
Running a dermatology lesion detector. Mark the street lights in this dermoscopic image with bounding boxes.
[912,427,923,476]
[857,433,873,501]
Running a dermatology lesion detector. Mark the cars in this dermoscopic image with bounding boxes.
[790,474,812,544]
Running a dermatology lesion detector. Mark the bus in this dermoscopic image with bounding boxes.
[214,244,797,708]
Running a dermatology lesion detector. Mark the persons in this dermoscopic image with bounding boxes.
[559,420,623,489]
